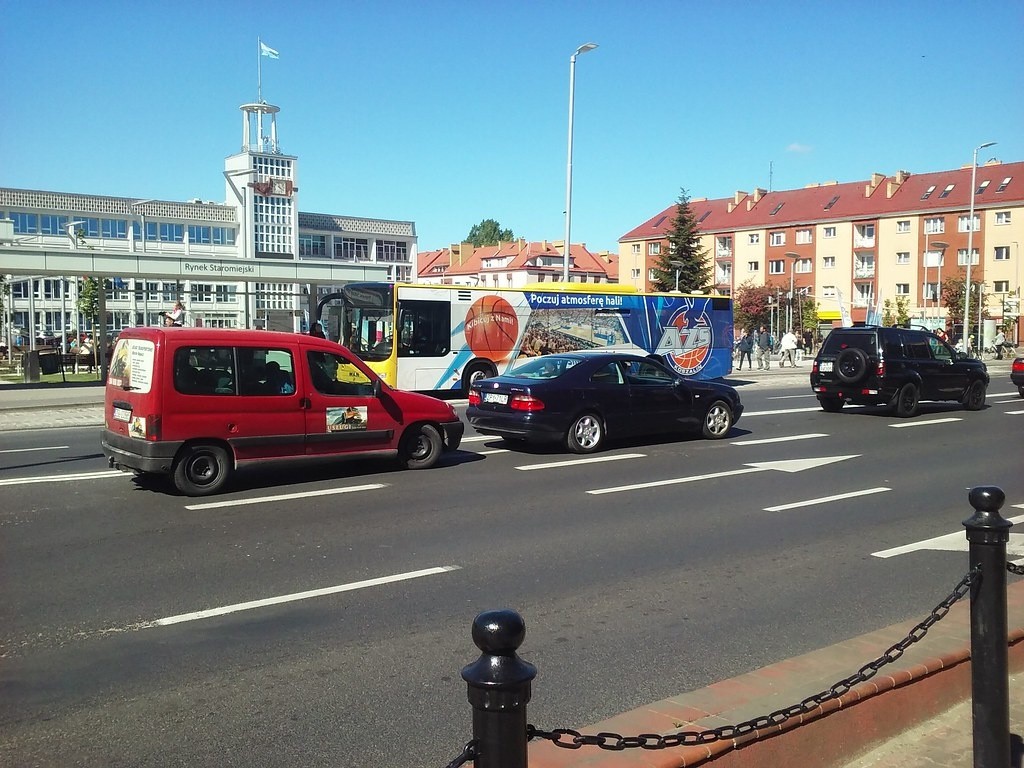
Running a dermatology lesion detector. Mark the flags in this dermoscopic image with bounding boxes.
[261,43,279,60]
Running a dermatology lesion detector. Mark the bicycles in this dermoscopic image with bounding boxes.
[982,339,1016,360]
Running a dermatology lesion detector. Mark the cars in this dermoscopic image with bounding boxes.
[1010,356,1024,396]
[465,350,745,454]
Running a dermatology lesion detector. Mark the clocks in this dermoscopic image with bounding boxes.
[273,180,286,194]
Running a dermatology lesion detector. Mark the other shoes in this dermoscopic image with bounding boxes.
[738,361,798,372]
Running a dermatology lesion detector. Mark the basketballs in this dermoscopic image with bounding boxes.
[464,295,518,363]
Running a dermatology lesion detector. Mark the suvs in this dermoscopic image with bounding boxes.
[810,322,990,418]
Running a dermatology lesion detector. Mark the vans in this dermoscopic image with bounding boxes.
[101,327,464,495]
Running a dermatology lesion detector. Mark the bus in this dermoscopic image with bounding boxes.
[310,282,733,399]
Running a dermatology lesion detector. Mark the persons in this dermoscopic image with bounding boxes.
[165,302,185,327]
[739,328,813,355]
[755,327,771,371]
[779,330,798,368]
[994,328,1004,360]
[60,332,119,355]
[937,328,948,347]
[736,329,752,372]
[953,335,974,357]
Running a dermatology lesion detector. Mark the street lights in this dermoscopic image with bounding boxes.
[563,41,599,283]
[930,241,948,326]
[671,259,683,294]
[962,142,999,353]
[784,252,801,331]
